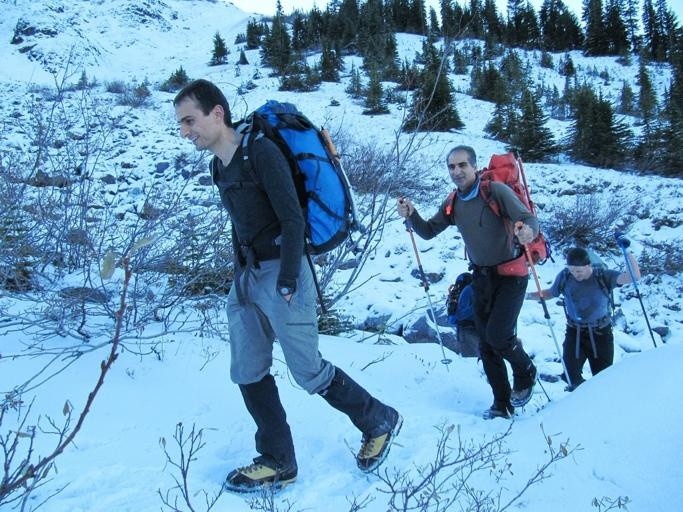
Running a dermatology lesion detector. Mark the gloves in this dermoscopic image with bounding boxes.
[616,234,633,255]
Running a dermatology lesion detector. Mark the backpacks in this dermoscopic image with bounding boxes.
[476,153,550,265]
[229,98,359,254]
[446,272,474,326]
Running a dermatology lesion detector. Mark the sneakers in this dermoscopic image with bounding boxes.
[483,407,515,419]
[355,406,404,471]
[225,459,298,491]
[509,367,539,406]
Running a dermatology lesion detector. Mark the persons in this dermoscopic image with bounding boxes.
[523,229,641,394]
[396,144,541,424]
[171,77,402,492]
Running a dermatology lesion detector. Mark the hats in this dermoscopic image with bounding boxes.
[567,248,591,266]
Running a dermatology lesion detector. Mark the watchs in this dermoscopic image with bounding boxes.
[277,286,295,296]
